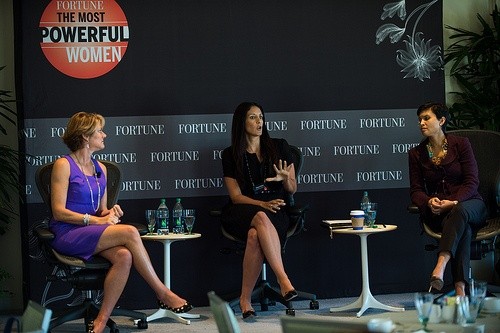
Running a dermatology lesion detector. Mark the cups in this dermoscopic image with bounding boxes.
[350,210,365,230]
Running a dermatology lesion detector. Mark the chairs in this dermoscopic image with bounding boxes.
[409,129,500,306]
[210,138,317,317]
[33,159,148,333]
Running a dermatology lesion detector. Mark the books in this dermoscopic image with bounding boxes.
[322,220,353,229]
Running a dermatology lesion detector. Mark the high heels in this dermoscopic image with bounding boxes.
[238,301,258,321]
[428,276,444,293]
[283,289,298,301]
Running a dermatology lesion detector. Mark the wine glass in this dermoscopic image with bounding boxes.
[413,278,487,333]
[145,209,157,236]
[364,202,376,228]
[183,209,195,235]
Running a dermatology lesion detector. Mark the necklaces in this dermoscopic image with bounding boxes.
[74,152,101,212]
[245,152,267,186]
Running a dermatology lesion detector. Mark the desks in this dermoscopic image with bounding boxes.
[330,224,405,317]
[133,231,202,325]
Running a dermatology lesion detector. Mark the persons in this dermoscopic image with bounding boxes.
[223,104,297,319]
[50,112,193,333]
[409,104,486,297]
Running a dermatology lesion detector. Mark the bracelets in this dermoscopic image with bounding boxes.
[83,213,90,226]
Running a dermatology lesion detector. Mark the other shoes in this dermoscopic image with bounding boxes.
[86,318,94,333]
[157,295,194,313]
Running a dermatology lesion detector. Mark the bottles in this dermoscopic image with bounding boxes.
[156,199,169,235]
[361,192,371,210]
[173,198,184,234]
